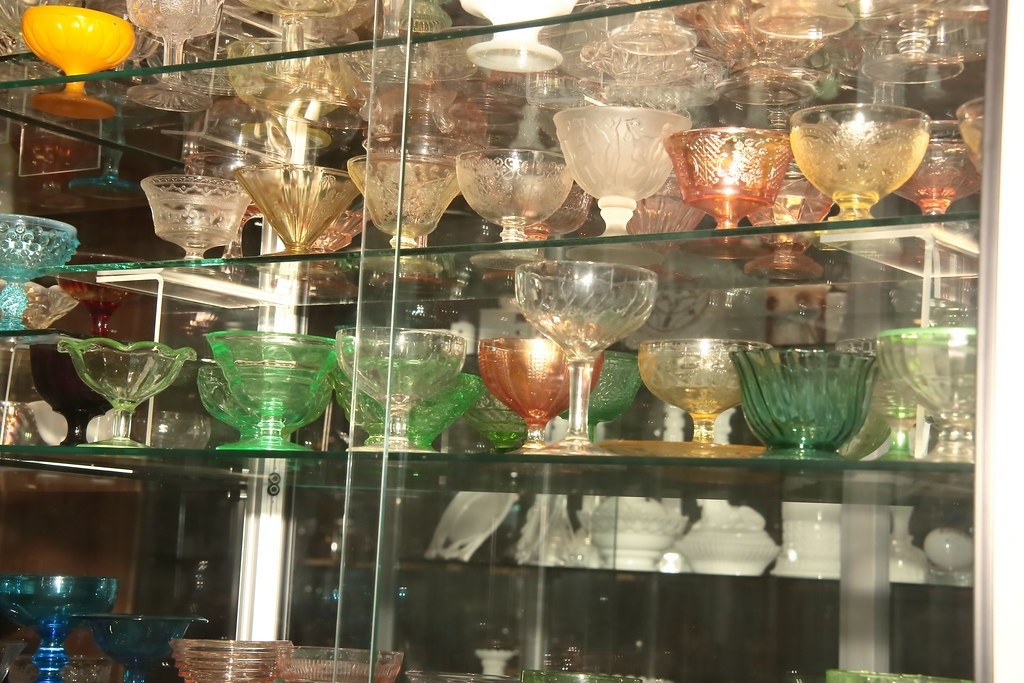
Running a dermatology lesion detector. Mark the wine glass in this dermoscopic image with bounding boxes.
[0,1,986,466]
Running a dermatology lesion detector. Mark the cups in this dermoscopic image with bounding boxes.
[825,668,973,683]
[0,572,645,683]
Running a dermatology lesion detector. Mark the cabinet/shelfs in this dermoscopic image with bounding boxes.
[0,0,1009,683]
